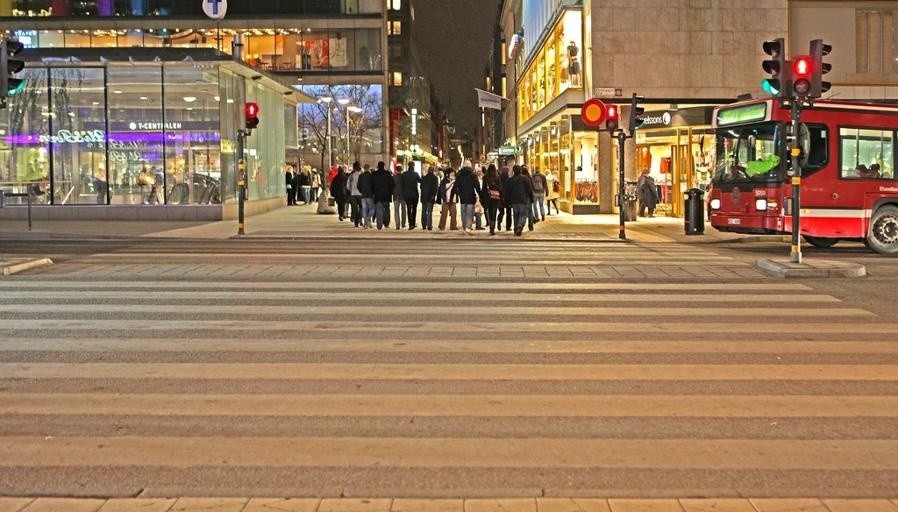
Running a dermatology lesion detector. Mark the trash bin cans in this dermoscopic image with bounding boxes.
[684,188,704,235]
[623,195,637,221]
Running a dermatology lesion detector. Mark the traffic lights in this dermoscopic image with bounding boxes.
[790,56,811,97]
[809,39,832,99]
[605,105,618,129]
[245,102,259,129]
[629,92,644,132]
[1,40,24,99]
[762,38,785,99]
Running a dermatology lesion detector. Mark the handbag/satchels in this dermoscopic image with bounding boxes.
[488,190,501,200]
[554,181,559,192]
[474,200,484,216]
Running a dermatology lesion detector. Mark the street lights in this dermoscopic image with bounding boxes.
[315,93,362,214]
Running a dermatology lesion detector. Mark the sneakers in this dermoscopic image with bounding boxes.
[476,224,512,231]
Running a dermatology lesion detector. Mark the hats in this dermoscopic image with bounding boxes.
[463,160,509,173]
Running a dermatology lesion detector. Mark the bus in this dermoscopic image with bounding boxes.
[709,97,898,255]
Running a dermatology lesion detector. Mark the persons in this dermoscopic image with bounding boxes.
[92,166,107,204]
[566,37,580,90]
[26,173,56,204]
[137,166,172,204]
[635,170,656,217]
[255,166,268,198]
[844,152,894,180]
[285,163,325,207]
[786,138,804,170]
[327,160,560,236]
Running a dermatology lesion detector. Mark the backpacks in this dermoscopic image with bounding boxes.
[534,174,544,192]
